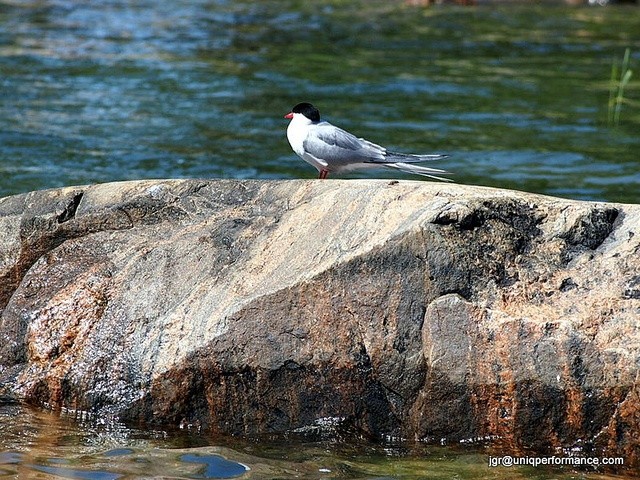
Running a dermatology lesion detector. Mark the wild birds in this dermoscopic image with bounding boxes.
[281,102,457,186]
